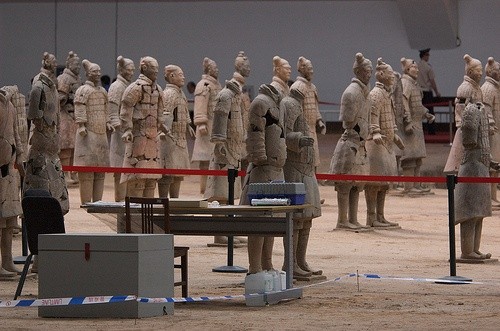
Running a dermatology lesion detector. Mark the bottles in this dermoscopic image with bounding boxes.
[244,267,287,294]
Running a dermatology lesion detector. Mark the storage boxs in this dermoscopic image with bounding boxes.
[36,233,173,320]
[170,199,208,207]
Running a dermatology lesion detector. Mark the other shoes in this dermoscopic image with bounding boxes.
[429,130,435,134]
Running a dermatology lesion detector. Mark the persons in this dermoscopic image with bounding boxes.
[191,57,222,196]
[56,52,84,184]
[100,75,111,93]
[283,56,326,277]
[269,55,293,99]
[238,84,286,279]
[186,81,196,133]
[328,53,436,230]
[23,129,69,274]
[28,52,61,139]
[443,53,500,260]
[0,85,24,277]
[74,59,115,207]
[7,85,27,234]
[232,51,250,88]
[106,56,193,202]
[418,48,440,133]
[205,79,248,244]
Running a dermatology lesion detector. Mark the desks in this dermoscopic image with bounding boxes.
[80,203,315,307]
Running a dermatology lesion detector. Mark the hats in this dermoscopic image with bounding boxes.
[419,48,430,54]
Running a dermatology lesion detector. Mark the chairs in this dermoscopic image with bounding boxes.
[123,197,190,302]
[13,195,65,299]
